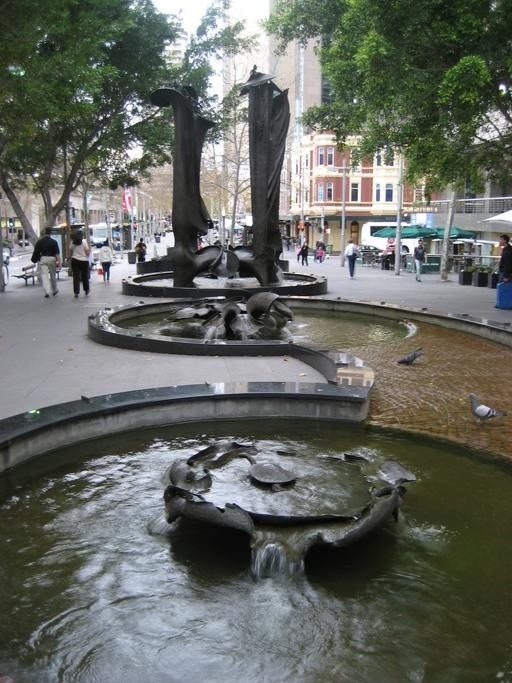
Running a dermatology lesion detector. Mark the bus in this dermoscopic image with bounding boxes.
[360,220,422,253]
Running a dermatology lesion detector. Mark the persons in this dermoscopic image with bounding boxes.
[495,234,512,308]
[98,241,112,281]
[285,238,324,266]
[345,238,362,278]
[414,240,428,282]
[31,227,63,299]
[134,237,146,263]
[197,236,246,251]
[69,228,90,297]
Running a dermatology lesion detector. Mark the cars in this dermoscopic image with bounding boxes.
[85,219,168,248]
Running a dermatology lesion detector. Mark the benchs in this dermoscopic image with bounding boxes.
[22,263,62,285]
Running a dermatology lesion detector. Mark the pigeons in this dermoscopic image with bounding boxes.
[467,393,508,424]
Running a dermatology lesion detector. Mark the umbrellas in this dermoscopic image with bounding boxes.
[217,218,253,234]
[373,227,476,269]
[478,208,512,227]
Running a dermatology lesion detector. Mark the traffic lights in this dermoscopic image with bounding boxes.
[8,217,14,227]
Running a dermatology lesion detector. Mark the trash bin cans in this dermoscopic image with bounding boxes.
[327,245,333,255]
[162,232,166,237]
[128,252,136,264]
[156,237,160,243]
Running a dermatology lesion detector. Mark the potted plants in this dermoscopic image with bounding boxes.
[459,256,500,289]
